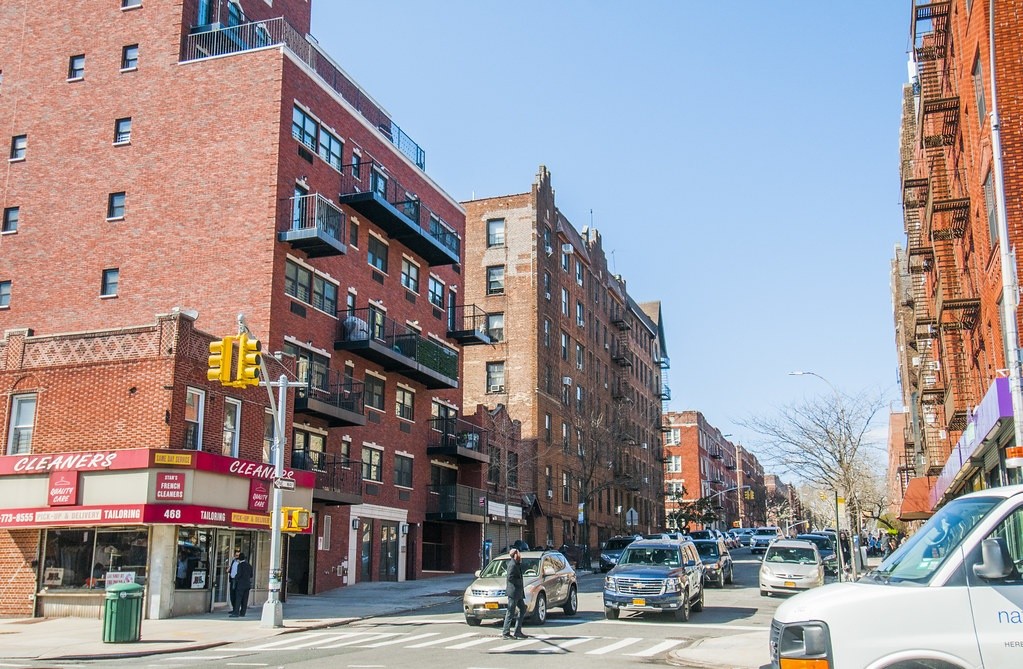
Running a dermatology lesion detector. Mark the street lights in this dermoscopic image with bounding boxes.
[788,370,857,582]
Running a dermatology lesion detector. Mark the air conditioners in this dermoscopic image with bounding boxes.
[491,384,501,391]
[642,442,647,449]
[563,376,571,386]
[546,246,552,253]
[912,357,918,365]
[934,361,941,370]
[546,292,550,299]
[928,324,938,333]
[562,243,573,253]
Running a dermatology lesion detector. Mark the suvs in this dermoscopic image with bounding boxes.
[602,534,705,622]
[464,549,578,627]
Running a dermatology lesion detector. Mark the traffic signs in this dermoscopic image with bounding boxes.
[274,477,296,492]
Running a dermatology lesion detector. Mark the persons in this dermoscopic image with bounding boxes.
[227,550,253,617]
[93,563,105,587]
[526,561,537,576]
[705,548,714,557]
[504,548,528,641]
[839,531,907,583]
[660,550,677,563]
[176,551,193,589]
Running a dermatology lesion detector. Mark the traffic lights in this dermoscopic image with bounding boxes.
[207,338,231,382]
[280,507,310,532]
[236,334,263,381]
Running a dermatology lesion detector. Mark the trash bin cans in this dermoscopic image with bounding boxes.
[860,546,868,566]
[103,582,146,643]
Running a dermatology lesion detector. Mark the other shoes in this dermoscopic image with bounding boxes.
[514,631,528,638]
[228,611,234,614]
[502,634,516,640]
[239,613,245,617]
[229,615,239,617]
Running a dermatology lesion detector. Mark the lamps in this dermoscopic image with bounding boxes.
[402,524,409,535]
[352,518,360,528]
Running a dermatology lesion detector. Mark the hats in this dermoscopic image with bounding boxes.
[509,549,517,558]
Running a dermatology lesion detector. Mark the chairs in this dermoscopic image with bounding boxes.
[771,551,784,562]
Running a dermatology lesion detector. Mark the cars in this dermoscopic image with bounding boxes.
[770,483,1023,669]
[759,529,835,596]
[600,527,785,588]
